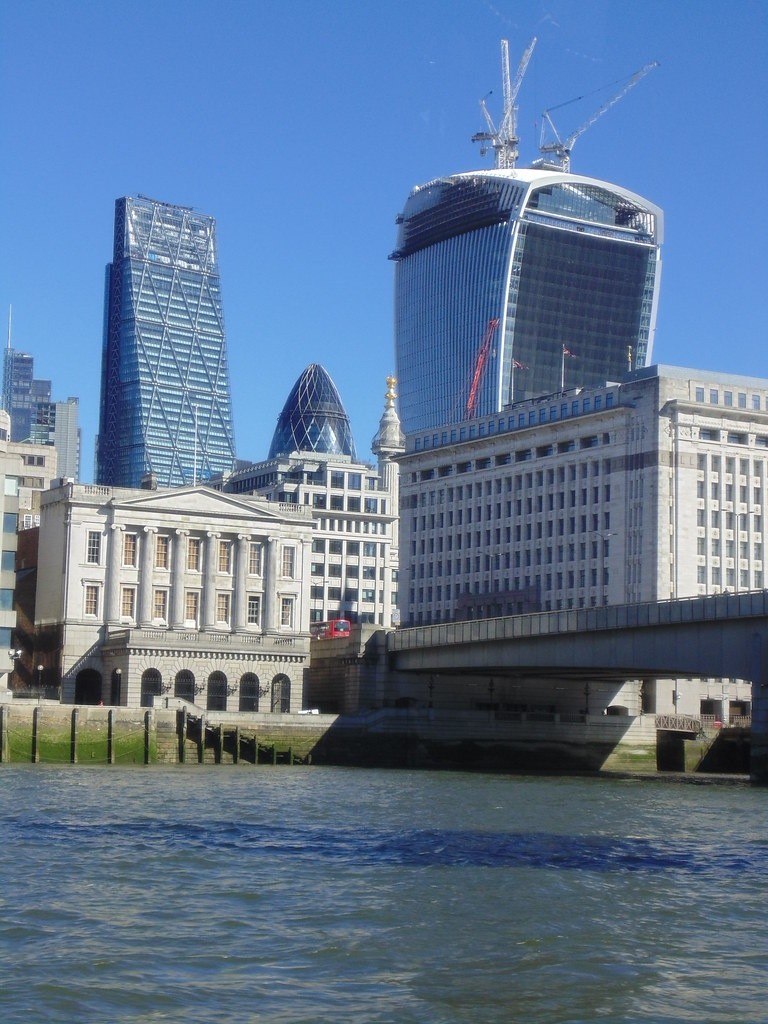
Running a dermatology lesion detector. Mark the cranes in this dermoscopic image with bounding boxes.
[529,60,661,174]
[471,33,540,167]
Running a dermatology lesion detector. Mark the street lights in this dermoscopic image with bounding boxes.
[37,664,44,705]
[587,530,618,607]
[116,667,122,706]
[720,507,755,594]
[477,550,504,592]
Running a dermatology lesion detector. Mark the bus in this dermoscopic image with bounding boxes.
[308,620,352,638]
